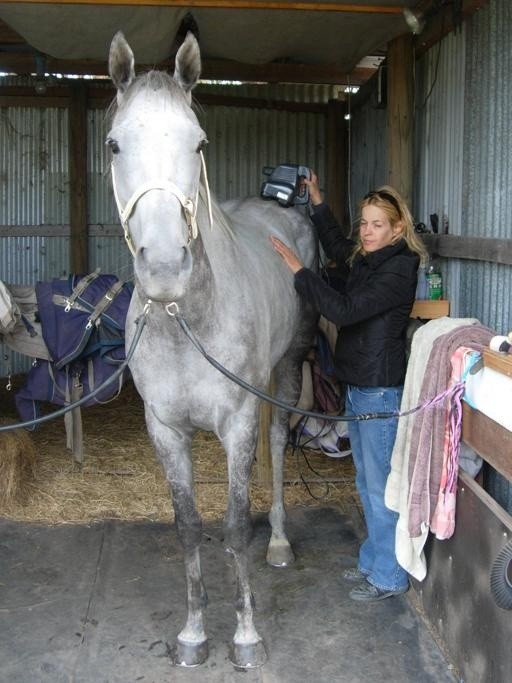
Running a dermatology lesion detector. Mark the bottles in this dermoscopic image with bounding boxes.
[427,256,442,301]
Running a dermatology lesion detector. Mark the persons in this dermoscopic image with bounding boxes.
[269,168,433,602]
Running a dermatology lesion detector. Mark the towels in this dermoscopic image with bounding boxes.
[384,316,500,581]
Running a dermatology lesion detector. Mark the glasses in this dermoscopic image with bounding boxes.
[363,191,401,219]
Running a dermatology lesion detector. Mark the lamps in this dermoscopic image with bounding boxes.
[402,0,447,36]
[34,54,46,95]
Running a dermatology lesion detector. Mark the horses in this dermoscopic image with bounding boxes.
[100,27,325,670]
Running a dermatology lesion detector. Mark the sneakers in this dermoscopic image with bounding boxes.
[342,568,369,583]
[349,584,404,602]
